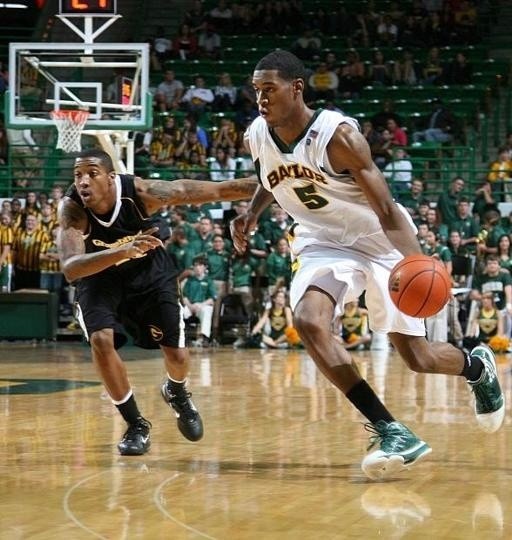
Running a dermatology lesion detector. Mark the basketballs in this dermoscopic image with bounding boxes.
[388,255,451,318]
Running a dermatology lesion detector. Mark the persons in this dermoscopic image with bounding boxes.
[57,150,259,456]
[0,0,512,353]
[229,50,505,480]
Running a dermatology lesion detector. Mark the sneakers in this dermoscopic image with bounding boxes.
[202,339,209,347]
[160,381,204,442]
[278,342,288,349]
[360,419,432,481]
[464,344,506,434]
[118,417,152,456]
